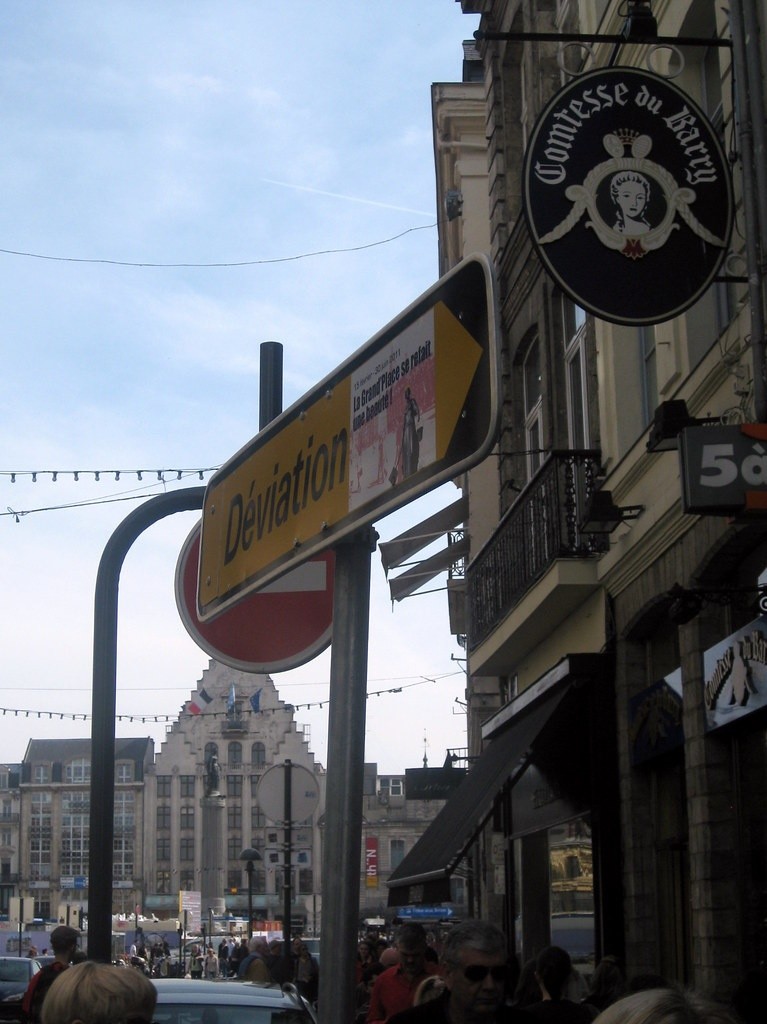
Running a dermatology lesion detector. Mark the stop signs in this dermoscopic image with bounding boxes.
[170,515,340,676]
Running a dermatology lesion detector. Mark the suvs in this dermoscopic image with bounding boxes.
[148,977,320,1024]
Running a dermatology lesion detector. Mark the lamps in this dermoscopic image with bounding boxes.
[646,399,729,452]
[576,488,645,534]
[617,0,660,44]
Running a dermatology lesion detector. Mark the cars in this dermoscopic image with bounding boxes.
[1,956,44,1004]
[31,956,58,969]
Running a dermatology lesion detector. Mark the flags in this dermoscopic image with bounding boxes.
[227,682,236,709]
[188,701,201,716]
[198,689,214,704]
[249,687,262,713]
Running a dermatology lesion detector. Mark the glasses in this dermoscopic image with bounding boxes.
[301,948,309,950]
[75,943,80,949]
[463,963,517,983]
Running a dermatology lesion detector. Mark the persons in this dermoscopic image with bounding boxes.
[208,754,222,791]
[398,387,421,479]
[0,925,766,1024]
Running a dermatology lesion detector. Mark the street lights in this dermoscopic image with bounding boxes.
[238,848,263,941]
